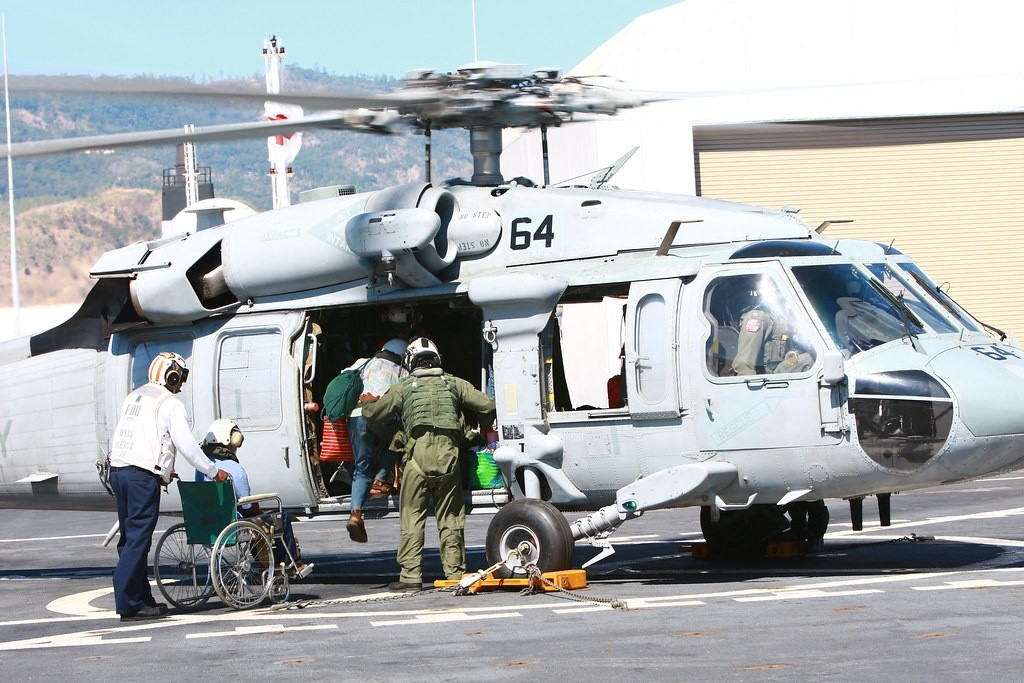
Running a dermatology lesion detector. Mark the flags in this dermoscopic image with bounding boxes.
[265,100,304,163]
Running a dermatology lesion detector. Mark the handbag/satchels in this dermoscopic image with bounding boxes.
[319,415,355,462]
[323,359,371,420]
[467,440,505,490]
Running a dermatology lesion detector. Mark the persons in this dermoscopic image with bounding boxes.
[194,418,303,575]
[358,336,495,592]
[732,278,814,375]
[112,350,227,620]
[340,338,410,543]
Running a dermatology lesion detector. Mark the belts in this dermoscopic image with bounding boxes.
[110,465,160,480]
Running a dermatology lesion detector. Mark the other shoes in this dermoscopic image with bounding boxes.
[287,564,304,578]
[258,566,269,571]
[121,597,168,621]
[388,582,422,593]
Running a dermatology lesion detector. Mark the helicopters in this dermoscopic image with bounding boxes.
[0,60,1024,583]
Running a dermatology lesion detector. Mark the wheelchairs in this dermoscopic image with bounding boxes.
[152,471,317,612]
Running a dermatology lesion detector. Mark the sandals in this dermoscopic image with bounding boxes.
[346,510,367,543]
[369,483,390,498]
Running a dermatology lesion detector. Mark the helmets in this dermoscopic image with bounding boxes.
[839,264,873,295]
[381,339,407,359]
[202,418,244,448]
[148,353,189,386]
[405,337,441,372]
